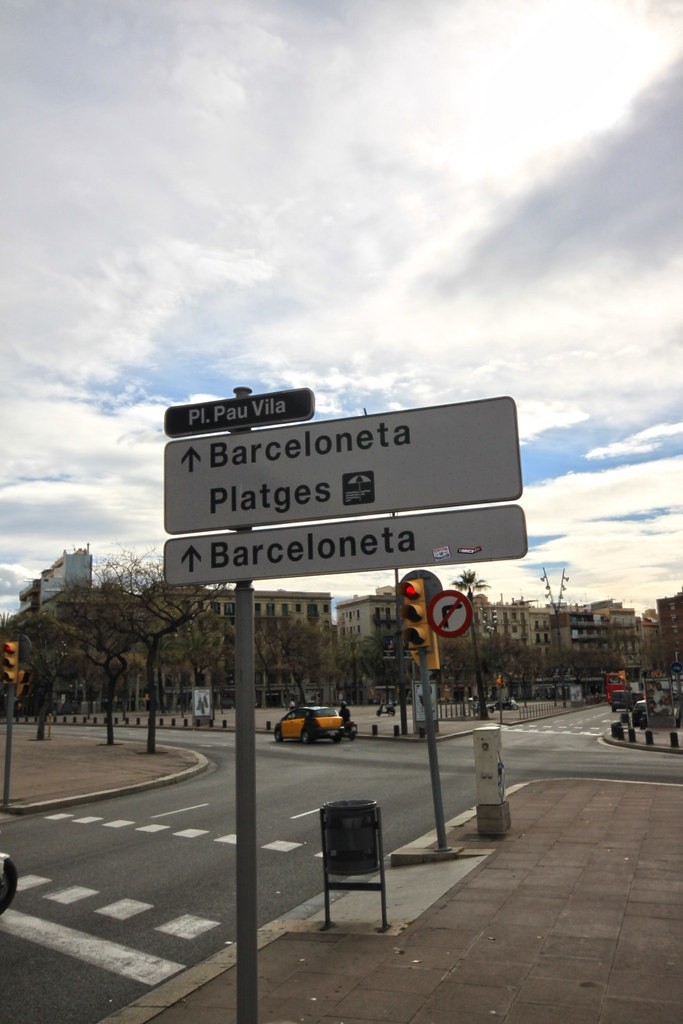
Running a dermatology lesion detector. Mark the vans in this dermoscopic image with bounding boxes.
[612,690,632,711]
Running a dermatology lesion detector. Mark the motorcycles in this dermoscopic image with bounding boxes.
[472,698,518,713]
[343,721,358,740]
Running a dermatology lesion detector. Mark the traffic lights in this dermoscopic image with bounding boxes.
[2,640,17,684]
[17,671,32,698]
[400,579,429,648]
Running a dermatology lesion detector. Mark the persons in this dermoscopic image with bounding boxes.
[339,701,351,722]
[289,699,296,719]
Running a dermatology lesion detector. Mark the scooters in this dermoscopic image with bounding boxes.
[376,701,397,716]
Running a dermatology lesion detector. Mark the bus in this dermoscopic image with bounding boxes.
[606,672,630,705]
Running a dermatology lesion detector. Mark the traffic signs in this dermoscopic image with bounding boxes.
[163,505,527,589]
[162,396,522,534]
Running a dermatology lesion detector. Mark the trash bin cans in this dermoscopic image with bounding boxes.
[620,714,628,723]
[324,800,380,875]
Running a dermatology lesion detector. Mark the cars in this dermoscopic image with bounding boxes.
[273,707,344,744]
[633,700,647,725]
